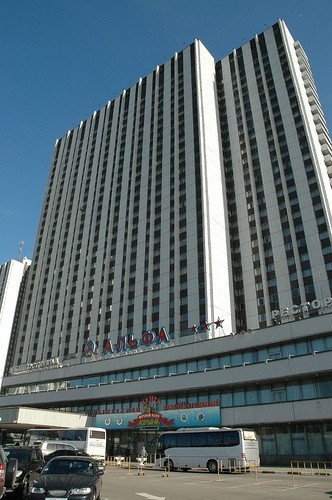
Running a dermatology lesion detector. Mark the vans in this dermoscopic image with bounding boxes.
[31,440,83,456]
[3,446,44,470]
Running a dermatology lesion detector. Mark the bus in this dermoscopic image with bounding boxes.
[23,426,107,462]
[153,426,260,474]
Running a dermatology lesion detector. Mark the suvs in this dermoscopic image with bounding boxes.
[0,444,19,498]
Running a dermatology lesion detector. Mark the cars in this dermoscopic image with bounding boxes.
[26,450,105,500]
